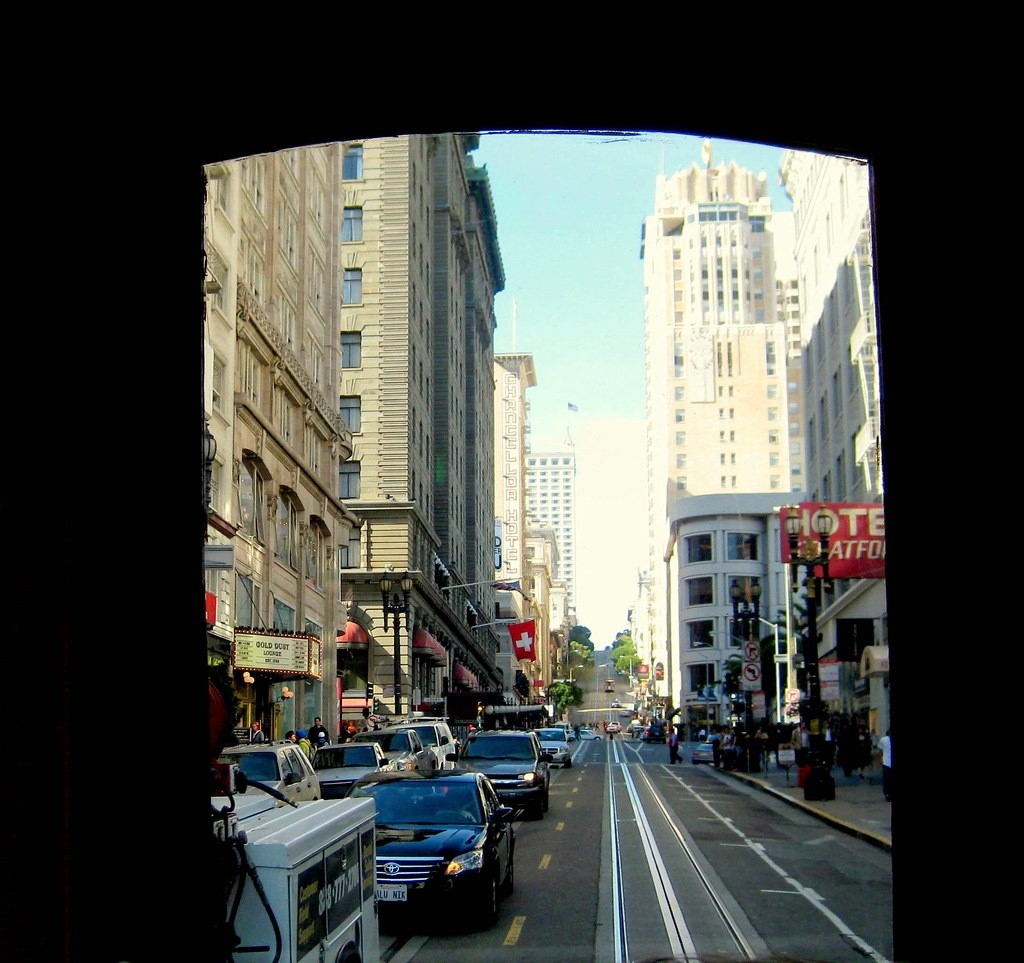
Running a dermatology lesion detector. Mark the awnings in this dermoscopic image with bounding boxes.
[861,645,890,678]
[336,620,370,649]
[411,627,478,690]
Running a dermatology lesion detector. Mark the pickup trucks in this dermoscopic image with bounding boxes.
[311,741,415,800]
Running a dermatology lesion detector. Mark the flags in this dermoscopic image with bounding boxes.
[489,581,533,603]
[509,619,537,662]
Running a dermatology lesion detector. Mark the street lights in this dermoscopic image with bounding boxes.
[569,665,583,684]
[379,568,413,715]
[709,615,784,746]
[620,655,633,687]
[730,577,763,773]
[682,641,725,734]
[567,649,578,668]
[782,504,837,800]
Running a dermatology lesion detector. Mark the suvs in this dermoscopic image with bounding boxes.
[445,729,554,820]
[346,716,461,778]
[643,726,667,744]
[217,739,322,808]
[531,728,572,768]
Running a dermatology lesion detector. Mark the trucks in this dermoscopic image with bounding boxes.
[210,796,387,962]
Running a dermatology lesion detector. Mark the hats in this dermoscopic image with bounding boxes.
[296,730,306,739]
[318,732,325,737]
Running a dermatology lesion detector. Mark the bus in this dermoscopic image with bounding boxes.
[550,721,569,730]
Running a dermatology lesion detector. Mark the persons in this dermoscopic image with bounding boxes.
[667,720,892,804]
[251,717,383,763]
[569,719,610,741]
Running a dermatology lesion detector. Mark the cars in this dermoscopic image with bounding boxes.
[344,770,519,931]
[604,678,643,734]
[565,730,604,740]
[692,743,714,765]
